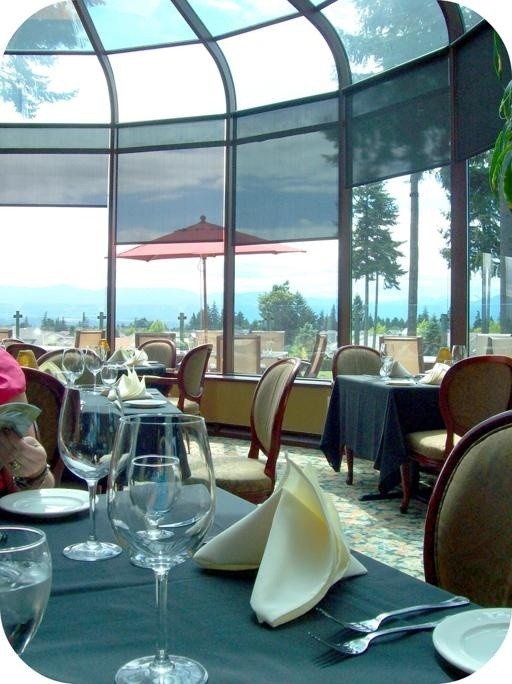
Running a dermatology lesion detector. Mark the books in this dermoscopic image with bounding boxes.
[0,401,43,436]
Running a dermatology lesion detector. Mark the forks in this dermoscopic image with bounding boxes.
[307,615,445,660]
[312,592,470,634]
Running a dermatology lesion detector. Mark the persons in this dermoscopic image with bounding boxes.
[1,346,56,498]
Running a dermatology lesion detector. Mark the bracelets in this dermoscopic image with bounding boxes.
[10,463,49,490]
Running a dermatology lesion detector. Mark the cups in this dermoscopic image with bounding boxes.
[0,524,53,657]
[55,369,74,389]
[442,360,454,367]
[451,344,467,363]
[101,364,119,387]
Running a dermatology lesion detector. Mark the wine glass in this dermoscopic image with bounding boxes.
[85,344,106,393]
[379,342,395,380]
[125,452,185,542]
[62,348,84,386]
[57,384,128,562]
[106,411,218,684]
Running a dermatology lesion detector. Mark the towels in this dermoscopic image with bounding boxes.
[198,457,367,626]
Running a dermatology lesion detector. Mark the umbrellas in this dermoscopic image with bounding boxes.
[102,214,307,344]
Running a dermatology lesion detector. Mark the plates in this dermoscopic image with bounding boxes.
[122,397,167,408]
[432,608,512,674]
[0,487,99,519]
[384,380,416,386]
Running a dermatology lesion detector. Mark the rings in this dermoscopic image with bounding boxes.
[7,460,21,472]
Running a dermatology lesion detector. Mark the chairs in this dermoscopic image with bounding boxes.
[189,360,302,504]
[19,366,72,479]
[419,410,512,607]
[398,353,512,507]
[4,318,512,409]
